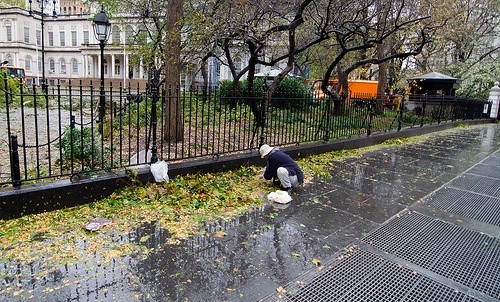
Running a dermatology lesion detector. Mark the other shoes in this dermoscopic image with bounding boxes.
[286,188,291,196]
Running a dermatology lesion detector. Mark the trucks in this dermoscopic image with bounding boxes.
[307,78,379,107]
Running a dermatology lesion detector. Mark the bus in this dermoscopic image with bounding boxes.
[0,66,25,92]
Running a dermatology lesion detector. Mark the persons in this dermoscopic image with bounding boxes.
[257,144,304,190]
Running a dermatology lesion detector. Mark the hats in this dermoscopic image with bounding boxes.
[259,144,274,158]
